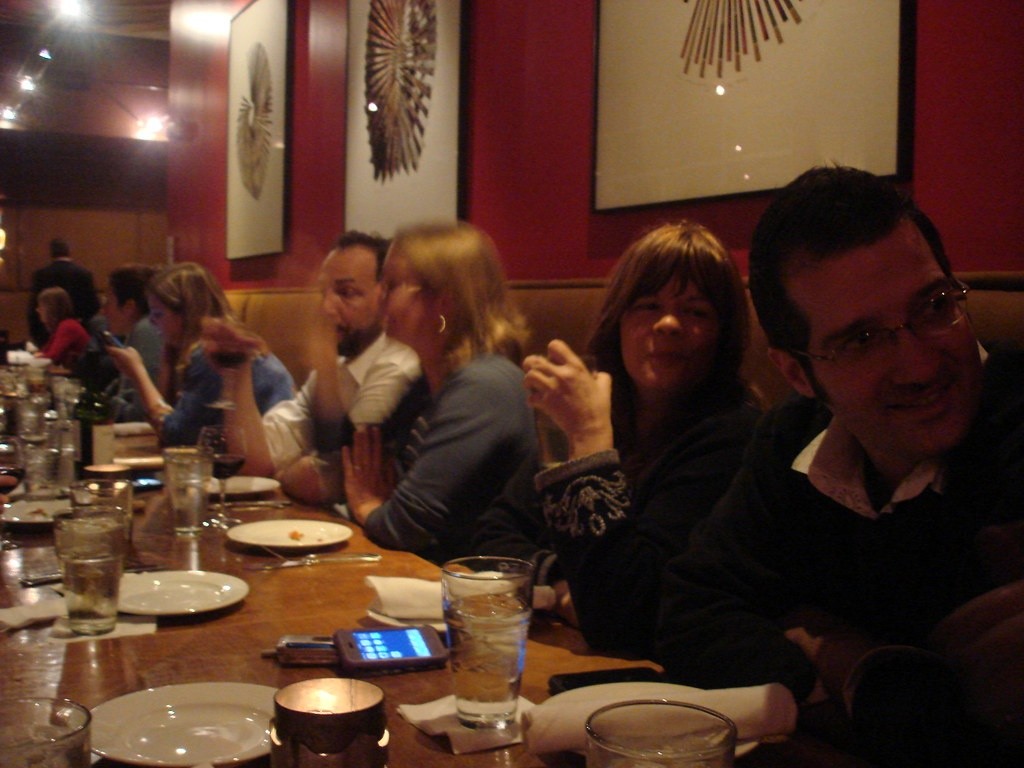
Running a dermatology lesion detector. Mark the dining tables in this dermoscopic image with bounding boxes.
[0,374,804,768]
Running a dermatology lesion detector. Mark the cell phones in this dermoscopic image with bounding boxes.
[102,331,124,349]
[549,667,663,697]
[261,635,336,666]
[334,625,451,675]
[131,478,164,490]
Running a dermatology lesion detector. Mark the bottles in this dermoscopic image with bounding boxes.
[43,410,59,449]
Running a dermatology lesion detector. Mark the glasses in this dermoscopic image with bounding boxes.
[781,279,970,376]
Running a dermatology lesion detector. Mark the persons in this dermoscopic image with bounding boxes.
[27,239,100,345]
[104,262,297,453]
[201,230,422,502]
[469,217,763,663]
[664,166,1024,768]
[36,287,92,359]
[341,223,536,561]
[98,265,163,408]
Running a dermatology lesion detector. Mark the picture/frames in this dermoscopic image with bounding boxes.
[343,1,471,240]
[589,0,912,214]
[224,0,293,261]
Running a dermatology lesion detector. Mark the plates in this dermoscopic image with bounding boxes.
[203,476,282,502]
[89,683,279,768]
[118,569,249,617]
[0,499,76,522]
[226,520,352,548]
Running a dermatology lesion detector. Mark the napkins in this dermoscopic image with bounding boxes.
[521,682,796,755]
[398,694,535,755]
[365,570,504,618]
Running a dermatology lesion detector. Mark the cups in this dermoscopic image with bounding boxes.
[537,355,598,469]
[441,557,536,730]
[53,505,132,636]
[69,479,132,510]
[272,677,385,756]
[51,378,80,431]
[585,700,738,768]
[1,699,93,768]
[26,445,59,500]
[83,464,134,498]
[26,368,47,400]
[16,399,46,442]
[163,445,215,540]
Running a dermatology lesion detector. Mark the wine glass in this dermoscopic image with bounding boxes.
[0,436,26,549]
[197,424,246,531]
[205,295,250,411]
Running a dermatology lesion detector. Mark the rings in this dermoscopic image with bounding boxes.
[354,465,362,471]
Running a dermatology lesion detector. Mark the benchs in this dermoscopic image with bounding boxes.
[225,269,1024,486]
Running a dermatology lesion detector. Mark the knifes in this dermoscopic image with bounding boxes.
[19,564,158,588]
[245,554,383,569]
